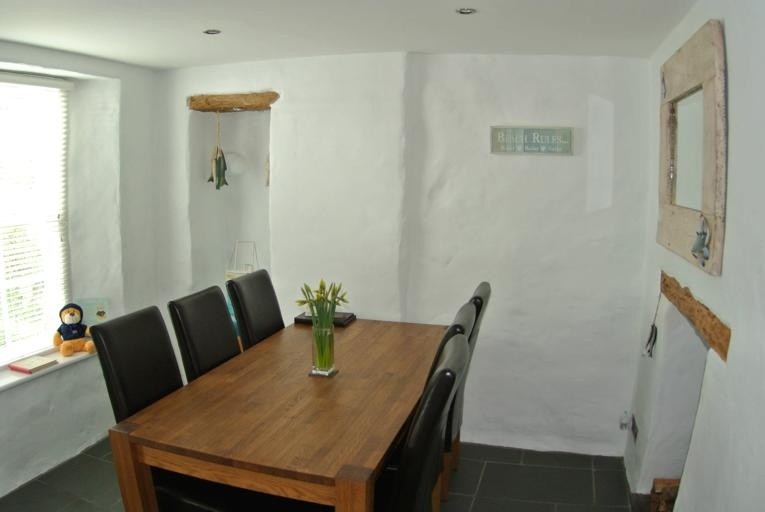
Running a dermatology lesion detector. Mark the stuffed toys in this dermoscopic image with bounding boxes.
[53,302,96,355]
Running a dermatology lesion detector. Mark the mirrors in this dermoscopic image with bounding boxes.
[654,17,726,279]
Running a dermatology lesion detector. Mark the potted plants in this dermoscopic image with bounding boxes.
[297,276,349,382]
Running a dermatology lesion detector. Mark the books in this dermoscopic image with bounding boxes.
[294,310,356,326]
[8,355,57,374]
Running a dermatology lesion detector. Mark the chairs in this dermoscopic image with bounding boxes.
[441,281,492,501]
[307,334,472,511]
[95,304,241,511]
[227,267,283,351]
[168,285,241,383]
[427,299,476,391]
